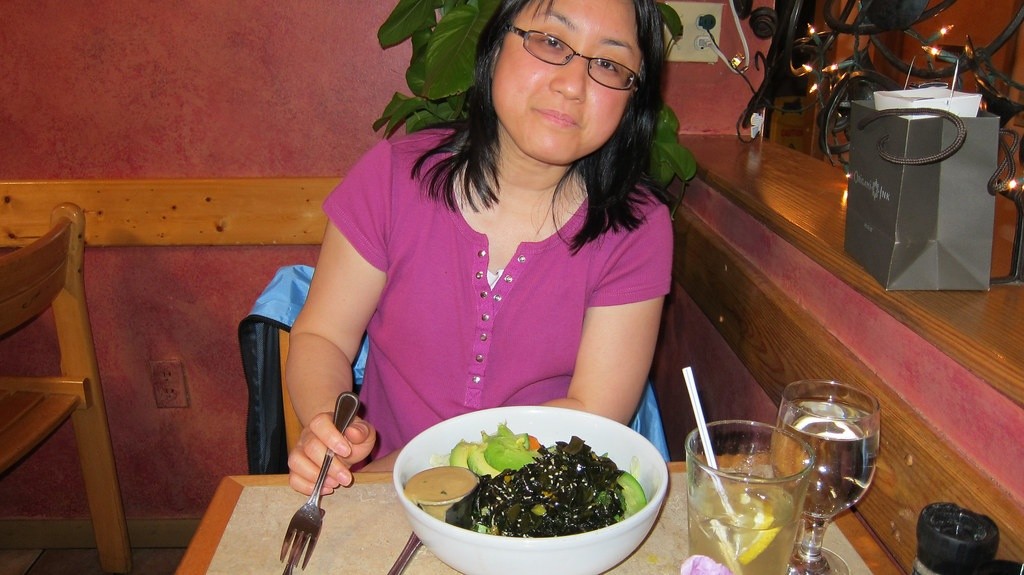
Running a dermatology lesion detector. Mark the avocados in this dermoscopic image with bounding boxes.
[448,425,540,481]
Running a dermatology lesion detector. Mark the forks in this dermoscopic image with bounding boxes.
[280,391,360,571]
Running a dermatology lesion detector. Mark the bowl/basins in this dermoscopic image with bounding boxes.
[393,405,669,575]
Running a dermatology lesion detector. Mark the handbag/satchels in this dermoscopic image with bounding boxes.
[843,99,1021,294]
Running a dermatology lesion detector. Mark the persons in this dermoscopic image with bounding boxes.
[286,0,673,496]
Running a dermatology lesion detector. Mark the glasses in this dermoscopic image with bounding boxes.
[506,25,641,90]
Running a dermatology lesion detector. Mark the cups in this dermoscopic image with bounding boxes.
[685,420,816,575]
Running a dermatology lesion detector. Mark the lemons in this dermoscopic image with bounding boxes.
[735,501,780,564]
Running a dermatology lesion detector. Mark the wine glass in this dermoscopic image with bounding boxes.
[772,378,881,575]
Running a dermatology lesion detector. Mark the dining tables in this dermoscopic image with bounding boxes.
[175,453,908,575]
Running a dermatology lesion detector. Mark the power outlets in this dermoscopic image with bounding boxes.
[662,2,723,62]
[150,361,188,408]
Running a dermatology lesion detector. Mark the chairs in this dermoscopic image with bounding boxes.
[1,202,134,575]
